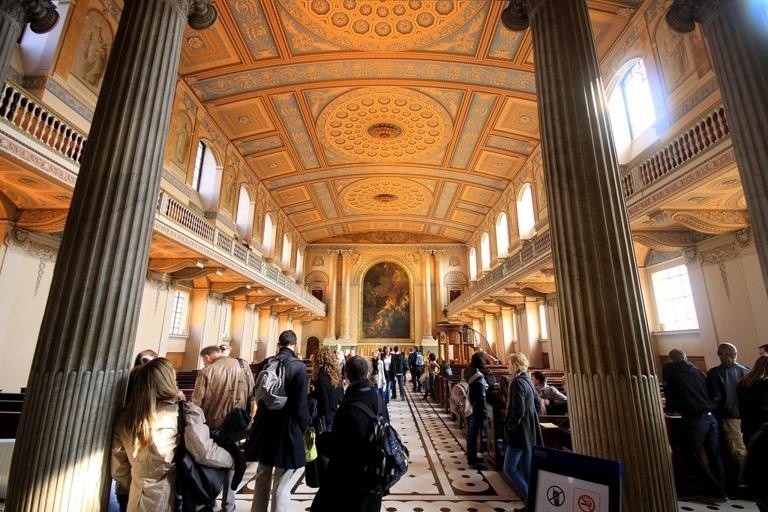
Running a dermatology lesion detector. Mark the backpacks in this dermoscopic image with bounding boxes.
[450,368,483,417]
[255,357,298,409]
[349,393,409,496]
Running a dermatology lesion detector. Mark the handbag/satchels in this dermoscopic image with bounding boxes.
[304,429,317,463]
[174,447,226,503]
[534,394,546,415]
[227,408,249,442]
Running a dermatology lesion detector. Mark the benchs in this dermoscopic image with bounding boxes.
[428,364,690,501]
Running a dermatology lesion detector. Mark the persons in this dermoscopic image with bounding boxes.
[464,351,568,503]
[661,341,768,512]
[111,331,390,512]
[370,346,452,401]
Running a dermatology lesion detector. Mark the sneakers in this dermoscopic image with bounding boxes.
[470,458,487,470]
[391,392,406,401]
